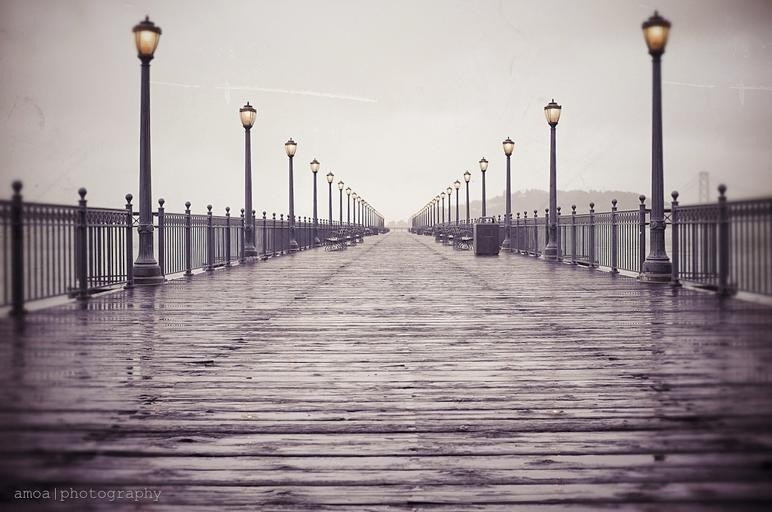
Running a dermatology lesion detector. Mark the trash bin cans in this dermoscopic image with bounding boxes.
[473,216,499,256]
[373,228,378,235]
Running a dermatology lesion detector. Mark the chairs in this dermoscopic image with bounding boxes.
[431,222,475,250]
[322,222,366,253]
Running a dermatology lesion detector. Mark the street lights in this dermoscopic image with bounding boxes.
[309,155,323,248]
[478,154,489,225]
[635,7,680,285]
[325,169,387,236]
[411,169,473,234]
[239,99,262,264]
[542,95,567,260]
[501,134,517,253]
[283,134,301,251]
[128,9,167,287]
